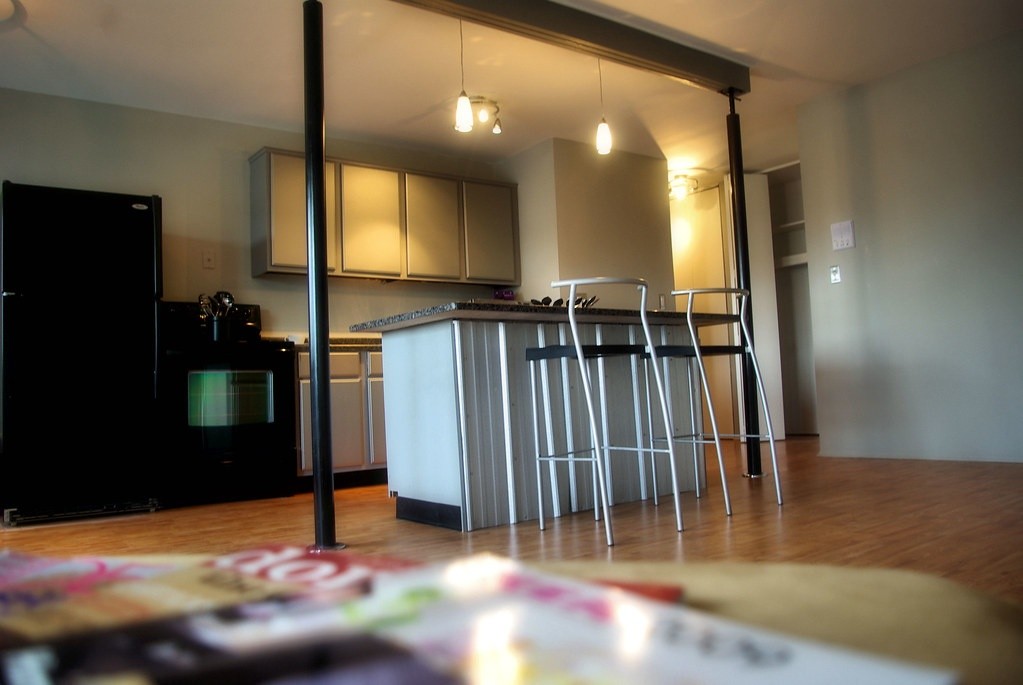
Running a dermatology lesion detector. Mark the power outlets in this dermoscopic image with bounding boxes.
[830,265,842,284]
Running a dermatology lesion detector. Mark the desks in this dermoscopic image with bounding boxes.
[527,556,1022,684]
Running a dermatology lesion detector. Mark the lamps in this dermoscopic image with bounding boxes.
[667,173,699,200]
[596,57,612,155]
[456,18,474,133]
[454,95,502,134]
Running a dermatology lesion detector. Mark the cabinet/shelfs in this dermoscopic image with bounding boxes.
[405,169,521,286]
[272,351,388,477]
[724,160,821,442]
[348,303,741,531]
[248,146,402,280]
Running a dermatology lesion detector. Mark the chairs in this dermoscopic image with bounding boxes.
[525,276,683,546]
[640,287,784,516]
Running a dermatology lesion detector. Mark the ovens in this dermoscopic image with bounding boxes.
[167,333,299,498]
